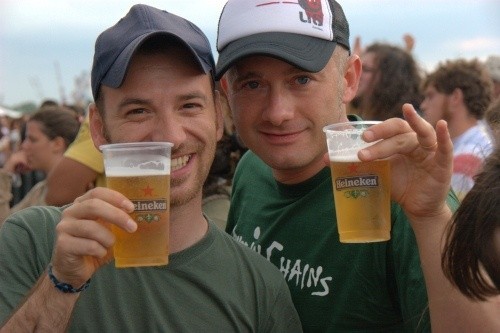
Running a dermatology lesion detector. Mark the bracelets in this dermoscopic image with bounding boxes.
[49,265,90,294]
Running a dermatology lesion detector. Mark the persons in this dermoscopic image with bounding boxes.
[0,4,307,333]
[0,45,500,333]
[199,0,500,333]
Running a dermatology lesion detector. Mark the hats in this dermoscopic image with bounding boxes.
[214,0,352,82]
[90,3,216,102]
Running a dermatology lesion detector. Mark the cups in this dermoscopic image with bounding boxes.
[100,142,173,268]
[324,121,392,243]
